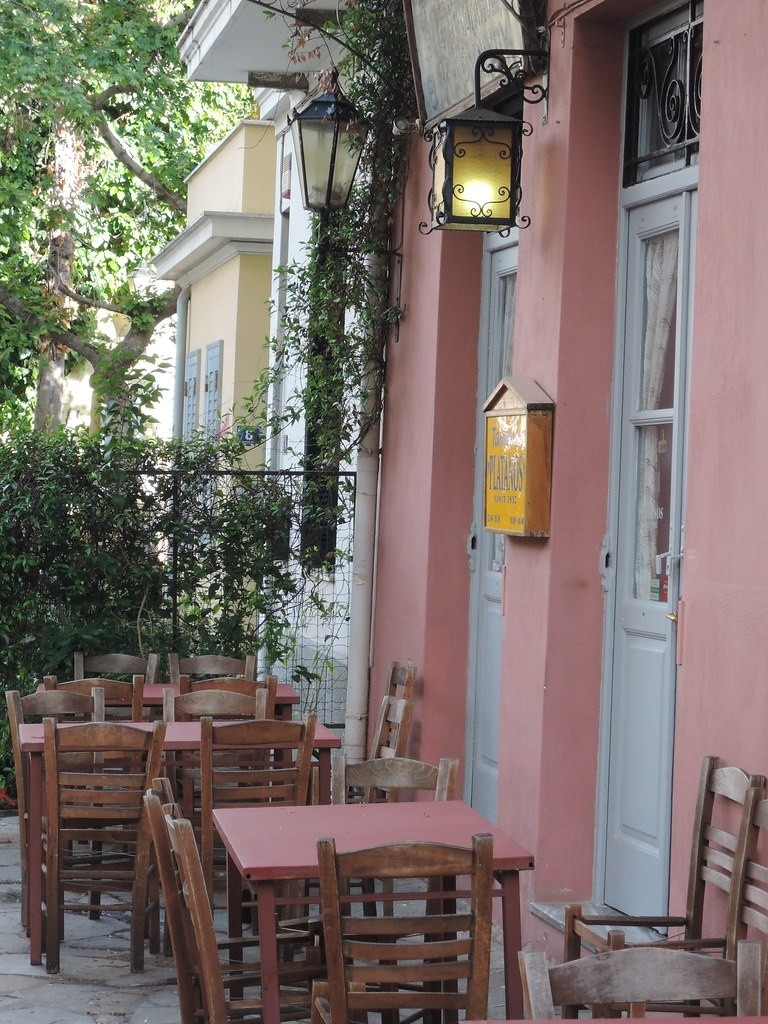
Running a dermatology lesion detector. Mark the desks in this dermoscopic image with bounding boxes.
[19,718,341,967]
[211,799,535,1024]
[36,682,301,802]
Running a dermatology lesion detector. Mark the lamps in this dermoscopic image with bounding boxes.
[418,27,551,238]
[286,64,406,344]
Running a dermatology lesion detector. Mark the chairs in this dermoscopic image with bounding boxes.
[0,651,768,1021]
